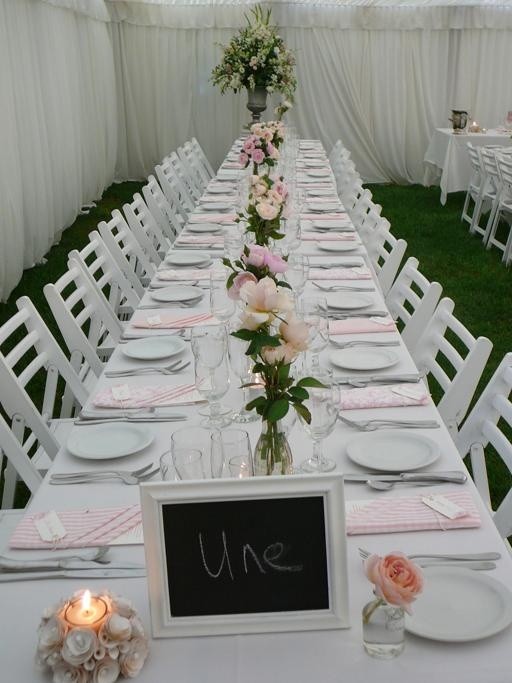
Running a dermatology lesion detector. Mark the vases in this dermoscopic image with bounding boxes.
[244,83,270,132]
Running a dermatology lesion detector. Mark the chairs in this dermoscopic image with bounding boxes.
[459,141,512,268]
[0,137,512,557]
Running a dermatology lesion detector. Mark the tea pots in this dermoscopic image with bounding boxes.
[448,110,469,133]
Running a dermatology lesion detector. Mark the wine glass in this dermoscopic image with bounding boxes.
[189,136,253,429]
[278,132,340,472]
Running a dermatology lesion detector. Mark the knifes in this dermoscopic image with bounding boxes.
[340,470,468,484]
[0,562,147,580]
[76,407,189,424]
[321,374,424,381]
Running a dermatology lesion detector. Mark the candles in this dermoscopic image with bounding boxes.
[59,590,111,632]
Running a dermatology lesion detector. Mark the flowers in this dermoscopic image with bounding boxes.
[359,548,424,615]
[206,4,296,97]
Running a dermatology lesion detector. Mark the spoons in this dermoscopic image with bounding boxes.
[1,541,113,564]
[366,478,403,494]
[120,328,186,340]
[149,280,198,287]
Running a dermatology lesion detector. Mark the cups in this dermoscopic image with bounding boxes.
[159,422,255,480]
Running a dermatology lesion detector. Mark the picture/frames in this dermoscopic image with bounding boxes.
[141,474,351,639]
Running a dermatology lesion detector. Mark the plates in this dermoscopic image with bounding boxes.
[343,431,439,470]
[312,203,373,309]
[64,422,154,463]
[167,222,223,269]
[153,285,202,302]
[329,346,398,369]
[382,561,512,643]
[123,336,186,359]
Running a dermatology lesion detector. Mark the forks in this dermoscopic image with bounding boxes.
[100,357,191,377]
[45,460,161,484]
[330,335,400,344]
[359,545,503,564]
[337,412,441,428]
